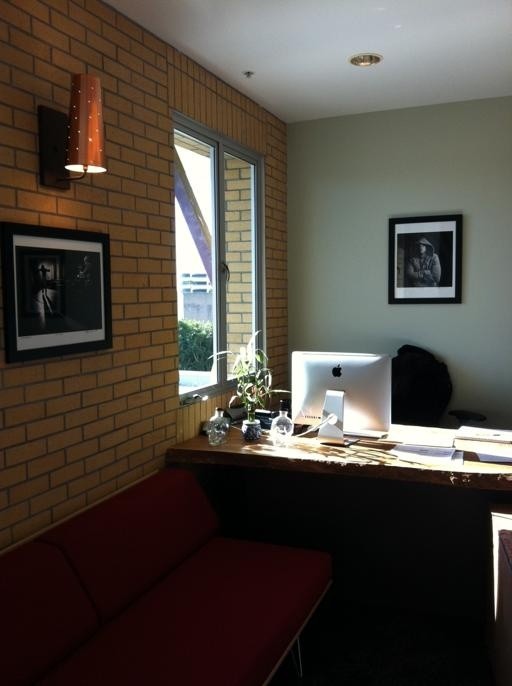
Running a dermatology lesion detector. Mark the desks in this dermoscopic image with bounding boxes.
[168,424,511,492]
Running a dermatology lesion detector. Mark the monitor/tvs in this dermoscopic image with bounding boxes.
[291,351,392,447]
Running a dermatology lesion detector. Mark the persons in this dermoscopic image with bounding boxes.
[38,264,52,294]
[405,236,442,287]
[80,255,97,291]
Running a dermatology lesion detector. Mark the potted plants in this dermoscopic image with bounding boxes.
[205,329,292,440]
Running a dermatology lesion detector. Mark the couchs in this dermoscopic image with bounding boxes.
[0,467,335,684]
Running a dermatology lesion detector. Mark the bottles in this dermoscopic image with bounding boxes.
[206,406,231,446]
[270,400,294,446]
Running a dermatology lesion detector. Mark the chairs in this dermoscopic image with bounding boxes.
[392,345,453,426]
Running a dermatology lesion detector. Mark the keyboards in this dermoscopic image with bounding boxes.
[342,431,388,440]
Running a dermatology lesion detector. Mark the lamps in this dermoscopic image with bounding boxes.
[37,72,107,190]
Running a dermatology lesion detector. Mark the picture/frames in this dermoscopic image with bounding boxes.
[0,220,113,364]
[388,213,462,304]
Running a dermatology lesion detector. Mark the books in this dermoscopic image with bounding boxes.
[454,425,512,444]
